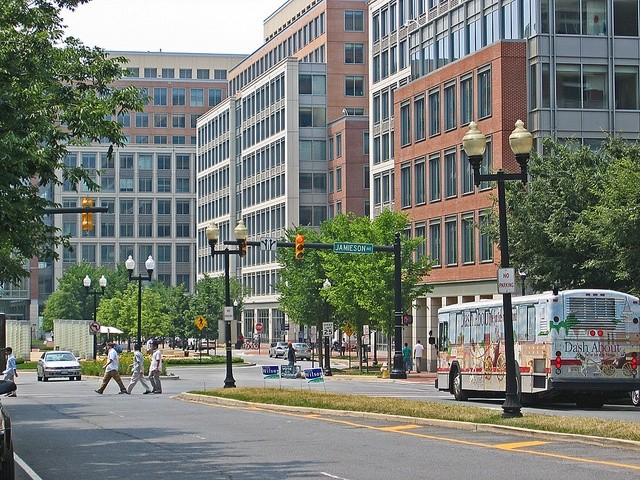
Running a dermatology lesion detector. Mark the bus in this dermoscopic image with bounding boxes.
[429,287,640,408]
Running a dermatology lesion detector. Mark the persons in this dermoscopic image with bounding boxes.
[341,338,347,355]
[287,342,296,365]
[148,341,162,394]
[122,344,151,395]
[403,342,412,374]
[414,340,424,374]
[94,342,126,395]
[2,347,18,397]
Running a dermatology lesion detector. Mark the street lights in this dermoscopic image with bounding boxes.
[125,255,155,351]
[462,119,533,418]
[83,274,106,359]
[31,324,36,351]
[206,220,247,388]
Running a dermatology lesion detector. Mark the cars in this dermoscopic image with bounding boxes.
[269,342,288,358]
[0,380,17,480]
[283,343,311,361]
[37,351,81,381]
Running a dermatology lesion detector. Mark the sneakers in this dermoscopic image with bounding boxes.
[4,392,12,396]
[94,388,103,394]
[125,391,131,395]
[143,390,151,393]
[8,393,17,397]
[119,390,127,394]
[154,390,162,394]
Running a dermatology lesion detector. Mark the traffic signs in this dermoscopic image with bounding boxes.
[334,242,373,254]
[323,322,333,337]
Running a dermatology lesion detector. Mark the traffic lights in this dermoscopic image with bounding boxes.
[403,315,413,325]
[82,198,92,231]
[295,235,304,259]
[241,240,246,257]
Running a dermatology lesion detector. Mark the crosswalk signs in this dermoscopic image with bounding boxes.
[195,316,207,330]
